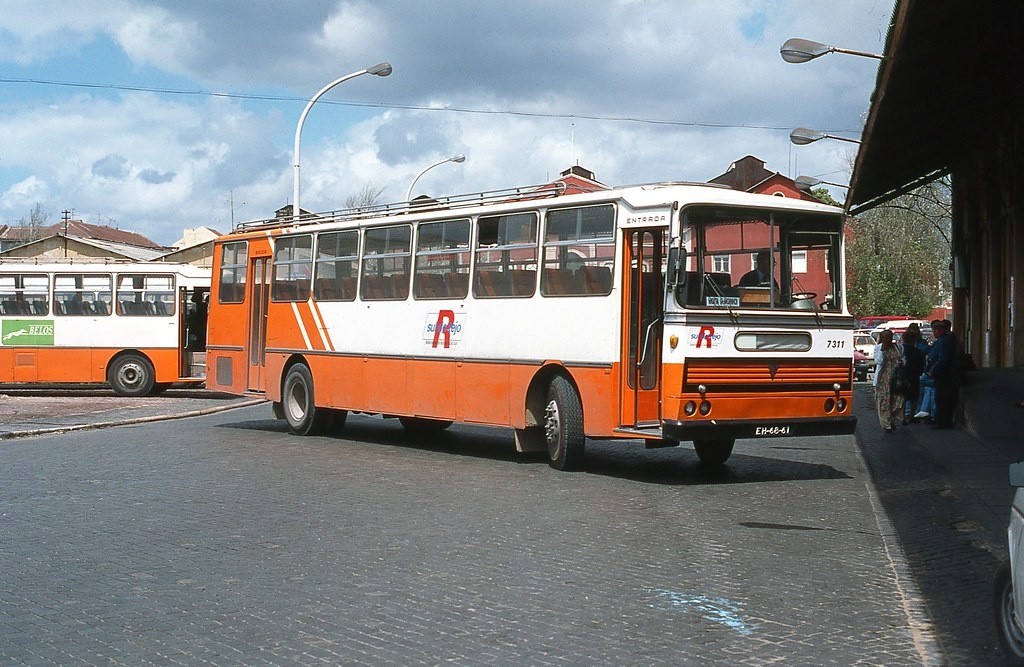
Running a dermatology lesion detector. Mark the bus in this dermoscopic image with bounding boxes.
[196,177,856,471]
[0,253,220,397]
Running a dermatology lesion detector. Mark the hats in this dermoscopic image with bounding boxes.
[931,321,945,330]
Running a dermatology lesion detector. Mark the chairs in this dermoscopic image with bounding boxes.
[1,300,168,316]
[219,266,731,309]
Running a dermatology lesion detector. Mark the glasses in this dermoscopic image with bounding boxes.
[762,260,777,266]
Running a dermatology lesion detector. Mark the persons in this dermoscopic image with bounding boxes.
[899,323,931,416]
[874,330,906,433]
[927,322,962,429]
[897,331,926,425]
[196,295,209,350]
[913,319,945,424]
[941,320,958,350]
[739,251,780,296]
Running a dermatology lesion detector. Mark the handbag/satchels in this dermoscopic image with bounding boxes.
[889,365,920,400]
[934,356,967,393]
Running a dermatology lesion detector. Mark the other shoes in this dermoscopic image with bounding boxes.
[884,410,955,432]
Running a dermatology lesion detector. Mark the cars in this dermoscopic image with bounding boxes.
[850,314,941,383]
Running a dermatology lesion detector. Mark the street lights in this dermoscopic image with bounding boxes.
[405,153,468,214]
[287,62,393,278]
